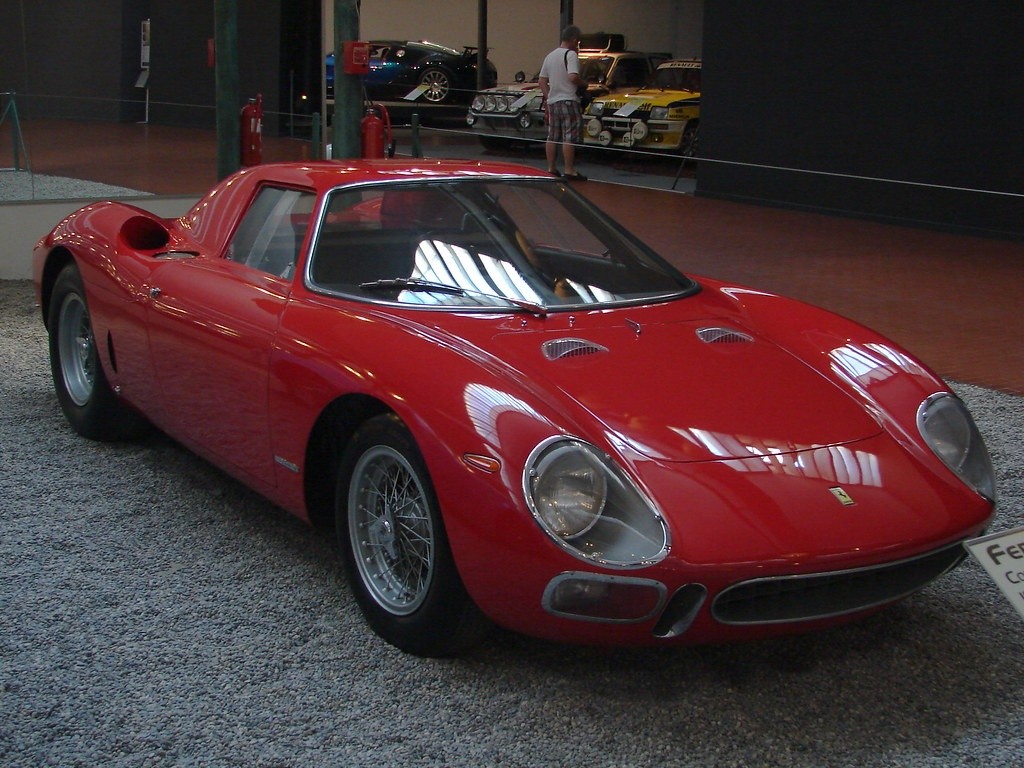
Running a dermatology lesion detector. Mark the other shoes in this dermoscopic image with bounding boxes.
[548,169,561,177]
[564,171,587,181]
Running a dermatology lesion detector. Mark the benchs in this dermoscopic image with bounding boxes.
[244,231,513,295]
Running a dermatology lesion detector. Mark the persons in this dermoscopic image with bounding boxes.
[538,26,588,181]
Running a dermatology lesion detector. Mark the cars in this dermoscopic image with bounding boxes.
[324,37,498,128]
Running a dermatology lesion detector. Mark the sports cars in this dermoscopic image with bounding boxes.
[31,156,1001,659]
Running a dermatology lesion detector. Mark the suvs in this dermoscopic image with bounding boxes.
[466,32,674,152]
[579,58,702,164]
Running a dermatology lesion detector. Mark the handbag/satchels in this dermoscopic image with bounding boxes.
[565,49,592,108]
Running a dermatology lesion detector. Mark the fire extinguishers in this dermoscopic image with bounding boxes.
[239,91,264,166]
[359,102,385,160]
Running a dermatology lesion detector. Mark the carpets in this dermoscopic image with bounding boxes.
[0,165,1024,768]
